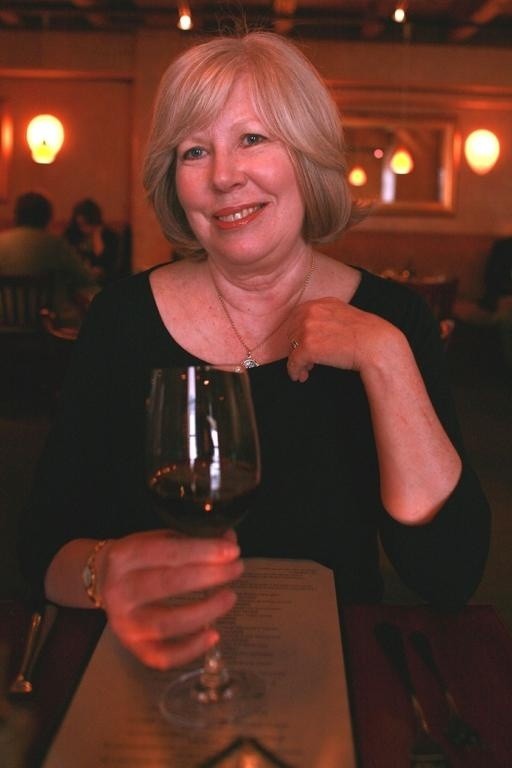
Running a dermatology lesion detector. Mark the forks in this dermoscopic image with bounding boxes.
[374,618,483,766]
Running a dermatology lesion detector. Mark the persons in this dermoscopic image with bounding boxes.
[63,195,116,268]
[22,21,488,669]
[481,228,510,345]
[36,237,106,325]
[1,192,104,309]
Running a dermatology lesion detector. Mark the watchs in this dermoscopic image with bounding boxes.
[82,540,104,609]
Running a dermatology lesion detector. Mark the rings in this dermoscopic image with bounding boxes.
[291,335,300,353]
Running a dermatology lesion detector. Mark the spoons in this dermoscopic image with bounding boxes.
[8,604,56,705]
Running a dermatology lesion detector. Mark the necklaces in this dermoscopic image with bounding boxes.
[203,262,308,369]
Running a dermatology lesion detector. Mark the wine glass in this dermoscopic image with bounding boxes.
[139,362,269,728]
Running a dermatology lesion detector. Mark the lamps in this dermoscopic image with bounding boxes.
[26,114,65,165]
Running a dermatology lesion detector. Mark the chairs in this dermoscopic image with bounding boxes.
[405,278,458,321]
[0,272,54,414]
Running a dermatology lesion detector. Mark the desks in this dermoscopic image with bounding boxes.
[2,600,512,768]
